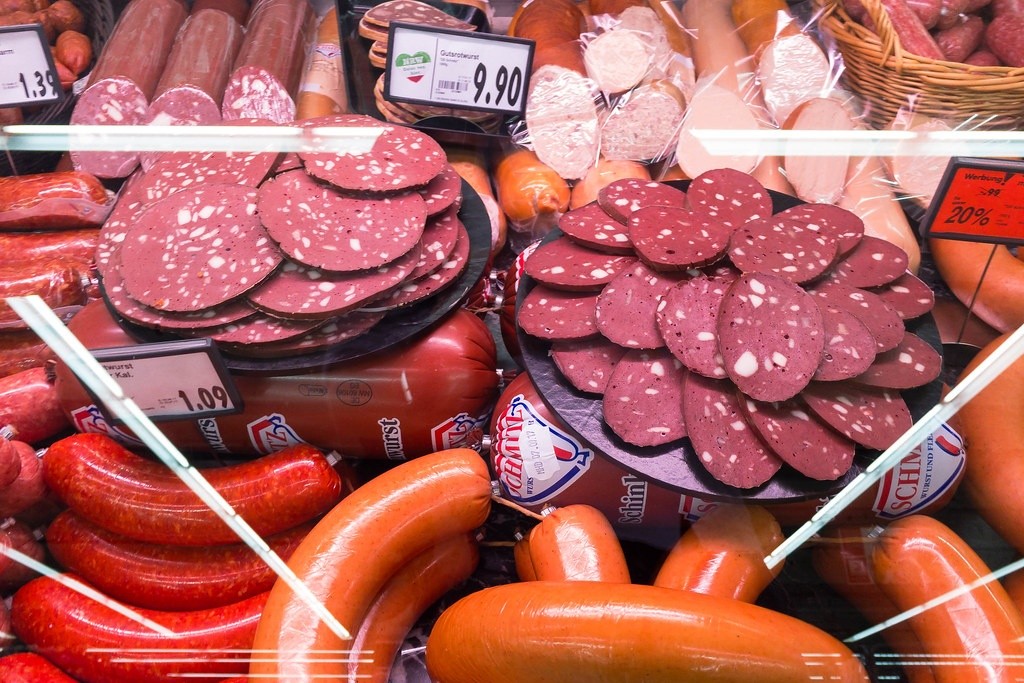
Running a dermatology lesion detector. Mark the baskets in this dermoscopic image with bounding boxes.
[812,0,1024,130]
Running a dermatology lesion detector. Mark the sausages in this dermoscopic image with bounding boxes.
[0,0,1024,683]
